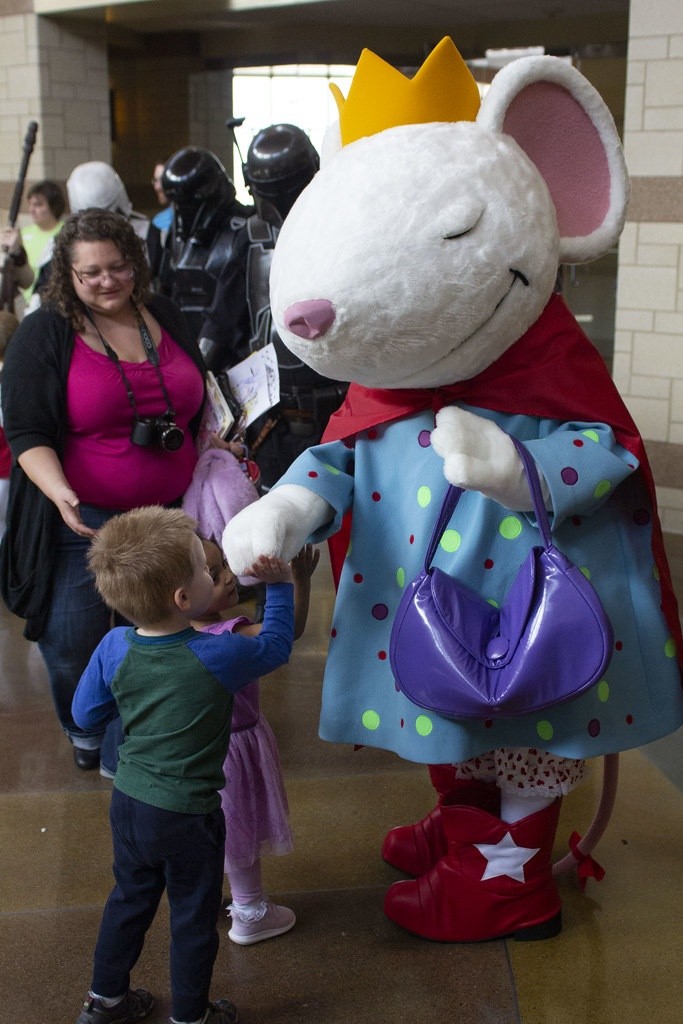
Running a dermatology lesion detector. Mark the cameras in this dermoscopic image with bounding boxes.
[129,417,185,451]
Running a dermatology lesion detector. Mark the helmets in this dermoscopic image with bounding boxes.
[247,123,322,194]
[65,160,132,221]
[161,144,231,200]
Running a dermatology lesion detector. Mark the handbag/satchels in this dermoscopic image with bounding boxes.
[385,431,615,720]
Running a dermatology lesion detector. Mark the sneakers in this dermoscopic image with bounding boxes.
[226,899,297,945]
[76,987,153,1024]
[169,998,239,1024]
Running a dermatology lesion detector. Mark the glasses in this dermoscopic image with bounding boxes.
[71,263,136,286]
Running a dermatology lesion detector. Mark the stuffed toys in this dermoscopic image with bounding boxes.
[220,34,683,943]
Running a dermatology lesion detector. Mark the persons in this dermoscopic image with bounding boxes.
[1,207,213,786]
[164,529,322,943]
[146,144,255,405]
[70,505,297,1024]
[65,159,153,279]
[198,122,351,649]
[145,155,202,333]
[4,178,85,326]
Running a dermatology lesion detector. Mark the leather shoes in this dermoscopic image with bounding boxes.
[75,748,100,770]
